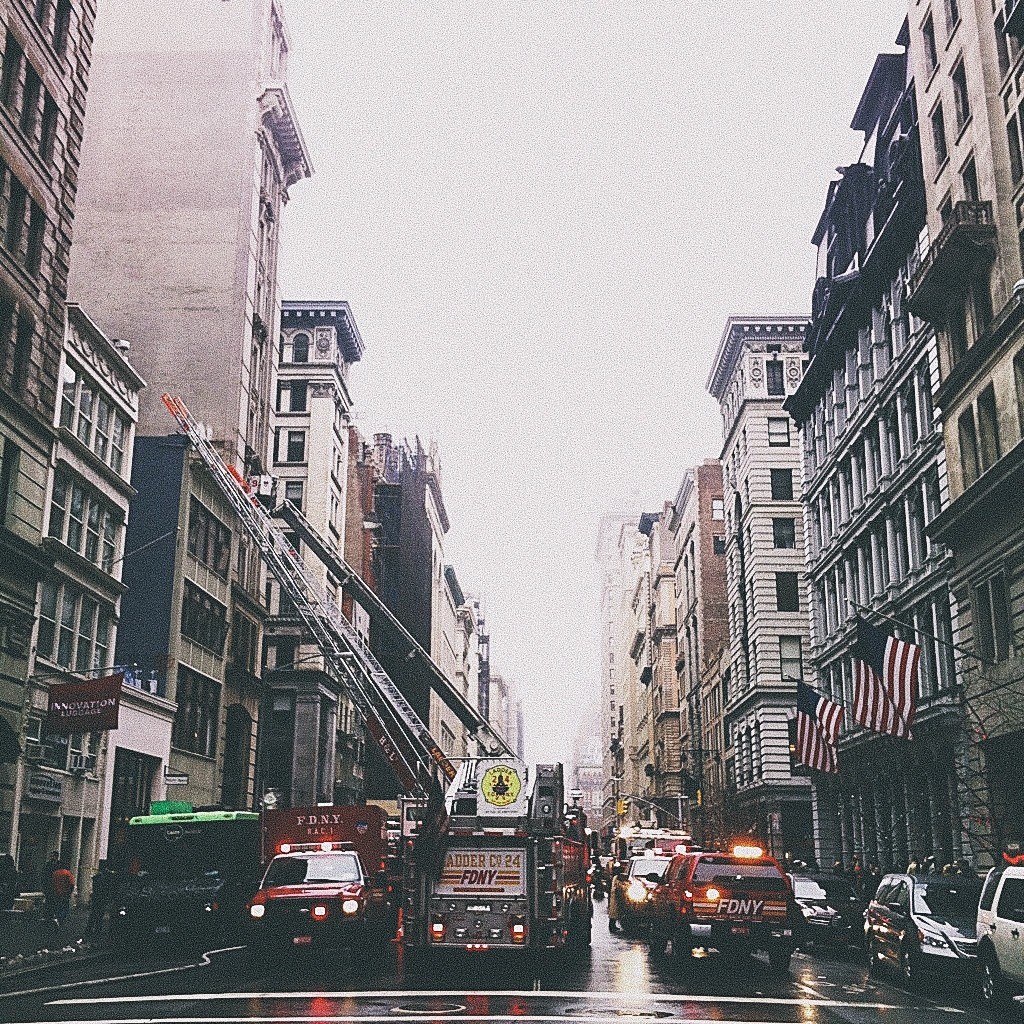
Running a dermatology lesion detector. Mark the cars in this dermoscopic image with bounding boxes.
[865,873,988,985]
[786,870,866,948]
[616,850,682,932]
[244,842,367,946]
[645,845,794,972]
[976,863,1024,1002]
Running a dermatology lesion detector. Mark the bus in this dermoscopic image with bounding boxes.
[116,807,260,945]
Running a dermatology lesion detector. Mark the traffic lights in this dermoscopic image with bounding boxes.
[617,798,629,815]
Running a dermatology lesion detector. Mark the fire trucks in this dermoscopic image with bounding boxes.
[165,396,601,966]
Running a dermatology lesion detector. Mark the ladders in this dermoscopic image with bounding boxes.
[159,391,520,812]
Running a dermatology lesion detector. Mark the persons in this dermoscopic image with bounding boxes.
[44,864,75,926]
[789,852,882,904]
[923,848,937,875]
[906,854,920,874]
[83,859,109,932]
[41,851,70,894]
[942,860,978,878]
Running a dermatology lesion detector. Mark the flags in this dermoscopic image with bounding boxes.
[796,683,845,773]
[850,615,922,740]
